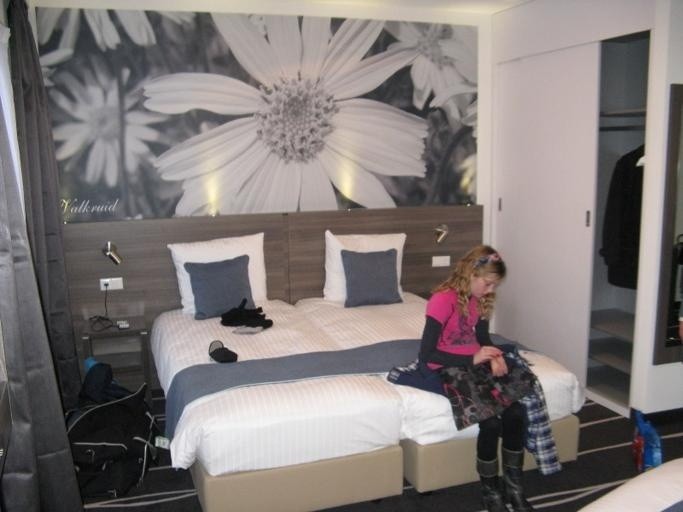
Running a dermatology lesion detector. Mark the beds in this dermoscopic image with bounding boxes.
[62,203,585,512]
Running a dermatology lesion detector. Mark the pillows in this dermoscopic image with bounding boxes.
[340,248,402,307]
[323,229,406,301]
[166,232,268,313]
[184,254,256,320]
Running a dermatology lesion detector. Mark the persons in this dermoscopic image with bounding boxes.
[418,245,533,512]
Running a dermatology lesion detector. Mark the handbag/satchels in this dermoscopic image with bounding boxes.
[64,363,159,499]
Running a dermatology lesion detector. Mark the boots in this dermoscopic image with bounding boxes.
[477,457,510,512]
[502,445,537,511]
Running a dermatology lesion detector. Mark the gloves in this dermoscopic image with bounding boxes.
[221,298,272,327]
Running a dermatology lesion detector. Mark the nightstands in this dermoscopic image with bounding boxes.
[79,316,153,411]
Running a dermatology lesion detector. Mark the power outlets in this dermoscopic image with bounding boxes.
[99,277,123,290]
[432,255,450,267]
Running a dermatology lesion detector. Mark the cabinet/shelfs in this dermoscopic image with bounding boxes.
[589,309,634,375]
